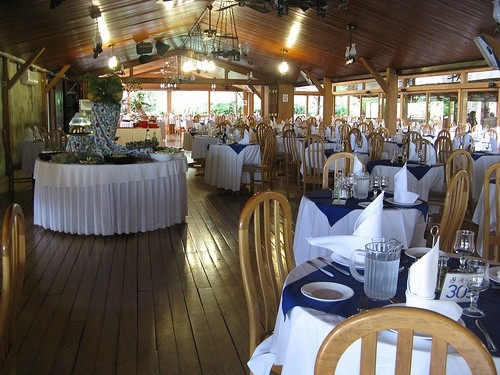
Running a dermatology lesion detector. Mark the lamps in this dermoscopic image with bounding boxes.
[88,0,357,76]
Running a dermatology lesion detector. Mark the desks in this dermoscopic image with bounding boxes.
[18,121,500,375]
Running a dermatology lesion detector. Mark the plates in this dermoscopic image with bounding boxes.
[385,198,423,207]
[404,247,445,260]
[382,303,466,339]
[489,266,500,284]
[331,250,365,270]
[300,281,354,302]
[358,202,385,208]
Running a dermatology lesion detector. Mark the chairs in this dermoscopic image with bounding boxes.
[119,113,499,375]
[0,204,25,375]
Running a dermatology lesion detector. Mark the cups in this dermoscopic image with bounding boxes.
[404,291,436,303]
[436,256,449,292]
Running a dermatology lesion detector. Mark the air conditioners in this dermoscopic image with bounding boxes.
[22,70,39,85]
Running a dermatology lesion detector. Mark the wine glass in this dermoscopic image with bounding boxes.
[334,172,347,204]
[347,173,357,198]
[380,176,389,200]
[454,230,474,272]
[370,177,379,200]
[461,260,490,318]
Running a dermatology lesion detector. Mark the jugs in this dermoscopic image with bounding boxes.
[349,238,404,301]
[353,170,370,198]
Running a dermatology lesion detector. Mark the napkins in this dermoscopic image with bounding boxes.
[304,188,386,264]
[357,134,370,153]
[406,236,463,321]
[408,141,419,162]
[349,133,355,150]
[233,129,240,143]
[392,163,420,206]
[427,144,436,165]
[327,127,332,141]
[226,125,230,133]
[353,155,363,175]
[334,128,341,141]
[238,129,251,145]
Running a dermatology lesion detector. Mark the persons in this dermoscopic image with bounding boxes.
[485,112,498,127]
[467,110,478,128]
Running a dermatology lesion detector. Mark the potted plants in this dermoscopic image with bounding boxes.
[91,70,127,151]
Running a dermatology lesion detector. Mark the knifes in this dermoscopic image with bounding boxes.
[317,257,350,276]
[307,260,334,277]
